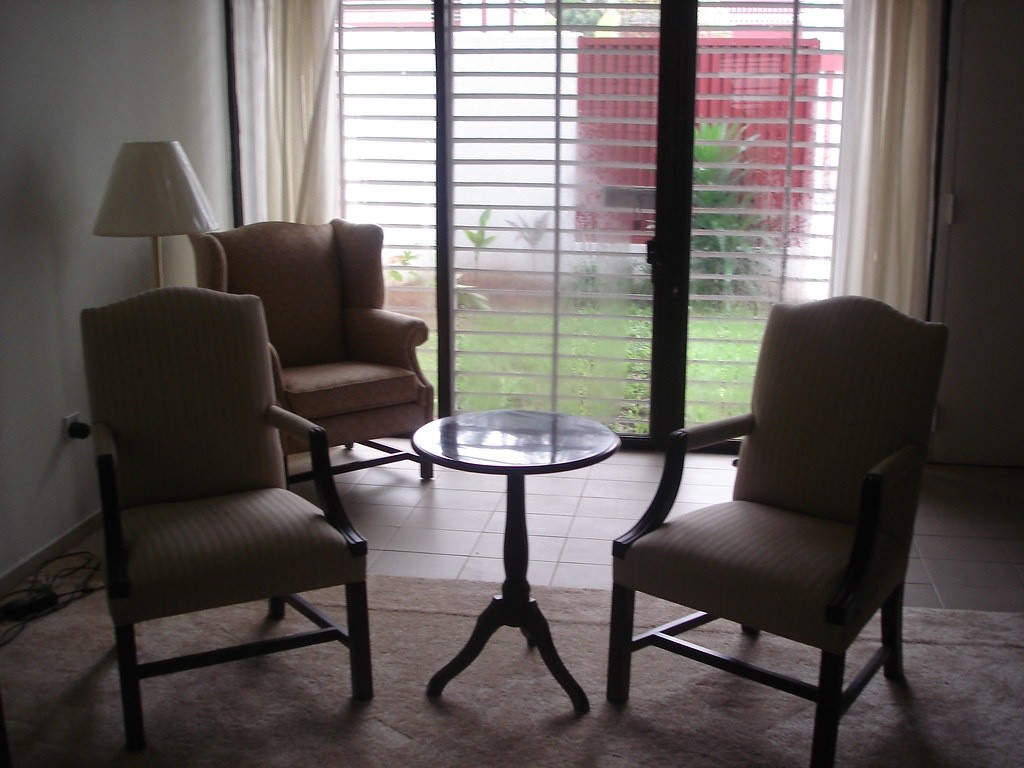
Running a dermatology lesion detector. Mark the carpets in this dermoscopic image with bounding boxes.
[0,550,1023,768]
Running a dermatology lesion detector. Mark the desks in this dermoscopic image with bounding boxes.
[412,410,622,714]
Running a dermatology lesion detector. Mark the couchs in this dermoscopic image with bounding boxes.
[188,217,433,480]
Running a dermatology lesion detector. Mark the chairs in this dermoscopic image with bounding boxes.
[606,296,948,768]
[80,287,373,754]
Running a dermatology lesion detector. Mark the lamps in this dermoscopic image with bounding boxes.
[94,140,219,287]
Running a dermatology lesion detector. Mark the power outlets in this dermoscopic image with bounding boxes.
[63,412,80,442]
